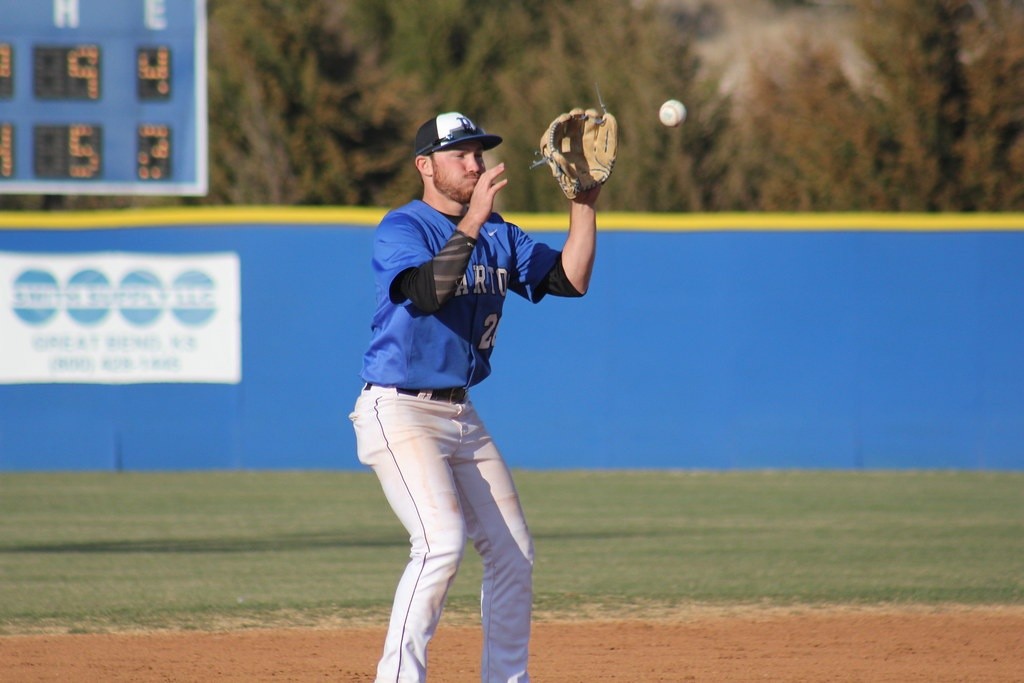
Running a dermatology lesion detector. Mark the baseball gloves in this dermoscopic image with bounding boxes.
[538,108,618,199]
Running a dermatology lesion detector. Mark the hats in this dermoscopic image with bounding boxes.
[416,111,503,156]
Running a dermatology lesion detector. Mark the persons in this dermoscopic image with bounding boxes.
[348,107,618,682]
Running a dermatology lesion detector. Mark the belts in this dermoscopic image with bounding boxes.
[397,385,467,403]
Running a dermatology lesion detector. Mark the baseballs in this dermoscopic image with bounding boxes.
[658,99,688,128]
